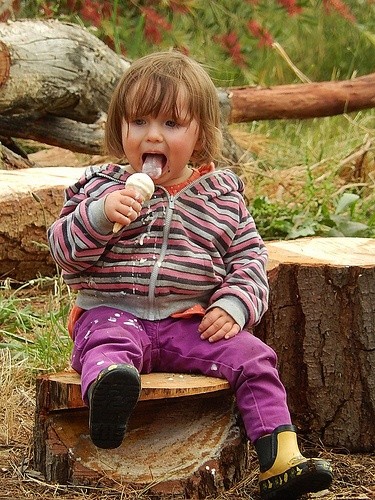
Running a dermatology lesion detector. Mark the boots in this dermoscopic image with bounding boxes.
[87,363,141,450]
[254,425,334,500]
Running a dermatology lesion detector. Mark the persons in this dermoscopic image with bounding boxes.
[46,53,332,500]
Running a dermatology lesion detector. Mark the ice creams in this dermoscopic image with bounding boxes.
[113,173,154,233]
